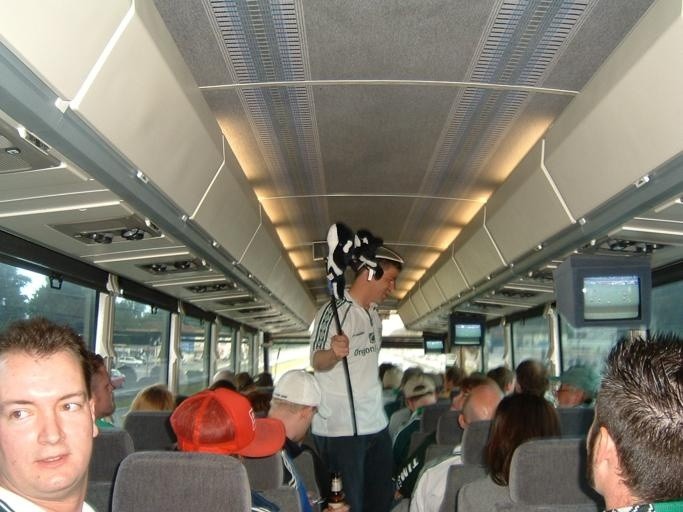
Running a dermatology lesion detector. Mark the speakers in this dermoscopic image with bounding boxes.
[313,241,329,262]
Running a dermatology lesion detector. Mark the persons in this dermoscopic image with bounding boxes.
[378,357,599,511]
[310,245,405,508]
[586,332,682,511]
[88,351,320,512]
[0,316,99,512]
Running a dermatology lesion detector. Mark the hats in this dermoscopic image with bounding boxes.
[349,242,407,274]
[166,386,289,462]
[380,364,467,399]
[557,362,601,399]
[274,365,335,420]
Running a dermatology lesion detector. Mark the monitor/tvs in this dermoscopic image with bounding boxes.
[423,337,451,354]
[447,314,485,346]
[552,254,651,329]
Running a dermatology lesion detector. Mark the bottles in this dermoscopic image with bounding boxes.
[328,471,346,509]
